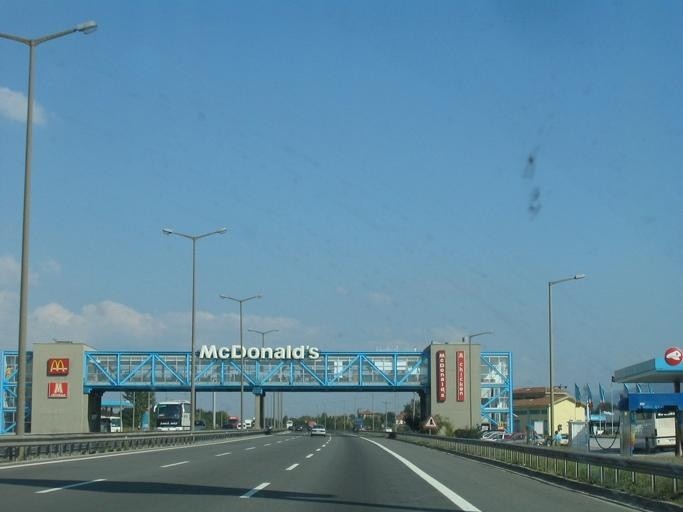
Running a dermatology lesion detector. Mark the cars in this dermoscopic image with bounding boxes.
[288,426,303,431]
[481,431,570,447]
[311,424,326,437]
[236,423,246,430]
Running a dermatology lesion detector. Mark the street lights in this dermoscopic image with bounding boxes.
[219,294,264,430]
[247,328,280,347]
[161,228,227,442]
[0,21,99,461]
[549,274,586,447]
[469,330,495,430]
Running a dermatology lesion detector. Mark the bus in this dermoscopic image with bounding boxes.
[99,415,122,433]
[619,411,678,453]
[153,400,192,432]
[286,420,294,430]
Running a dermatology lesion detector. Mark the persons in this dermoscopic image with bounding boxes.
[552,431,562,447]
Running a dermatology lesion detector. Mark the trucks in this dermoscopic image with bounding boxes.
[307,420,317,431]
[225,417,240,428]
[245,420,252,428]
